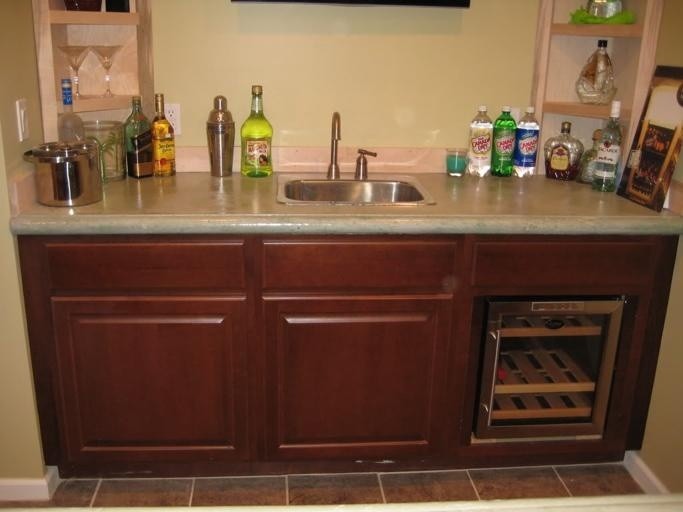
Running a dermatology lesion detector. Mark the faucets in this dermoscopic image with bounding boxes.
[327,112,342,181]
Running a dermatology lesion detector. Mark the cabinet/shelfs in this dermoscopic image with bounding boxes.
[33,0,155,145]
[530,0,663,193]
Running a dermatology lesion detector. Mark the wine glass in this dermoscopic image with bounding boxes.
[56,44,123,101]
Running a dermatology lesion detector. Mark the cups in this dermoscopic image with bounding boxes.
[444,147,467,178]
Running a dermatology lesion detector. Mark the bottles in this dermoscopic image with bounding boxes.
[467,100,623,194]
[238,83,274,179]
[59,79,178,181]
[204,95,236,181]
[574,38,619,107]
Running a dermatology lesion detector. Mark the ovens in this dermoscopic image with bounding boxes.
[469,293,639,443]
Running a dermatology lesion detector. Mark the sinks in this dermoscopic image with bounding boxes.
[275,175,437,207]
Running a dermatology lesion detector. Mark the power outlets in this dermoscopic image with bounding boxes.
[164,105,180,135]
